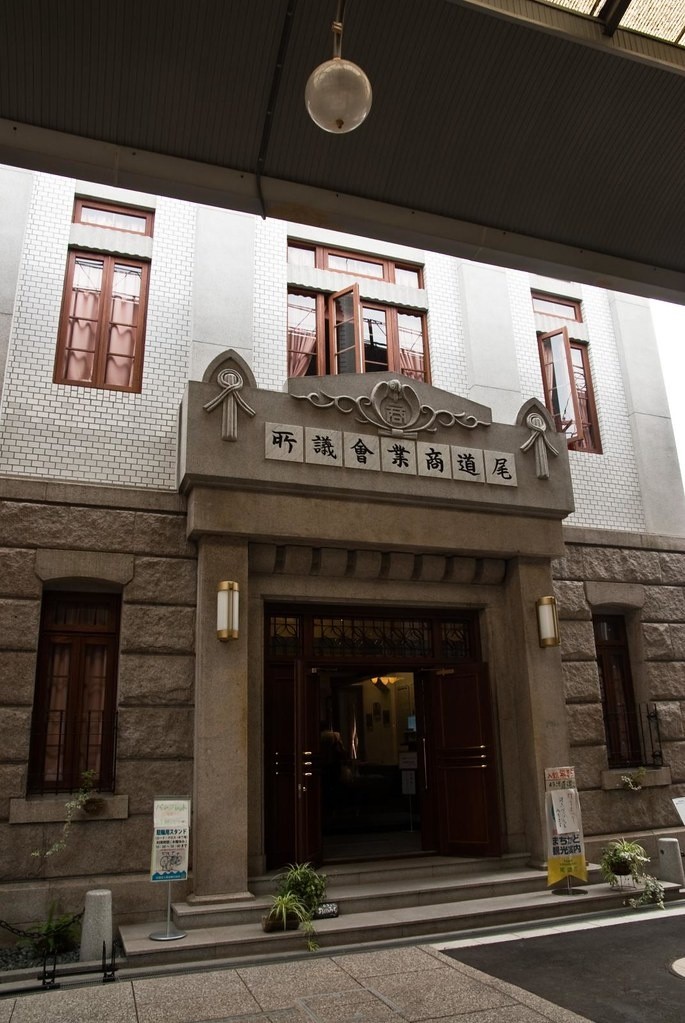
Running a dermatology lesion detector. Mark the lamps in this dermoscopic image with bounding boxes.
[536,595,560,649]
[215,581,240,641]
[303,2,371,134]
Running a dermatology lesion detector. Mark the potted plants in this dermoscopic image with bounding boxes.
[598,837,665,911]
[33,768,105,859]
[260,861,329,952]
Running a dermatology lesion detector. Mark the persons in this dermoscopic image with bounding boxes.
[316,718,344,818]
